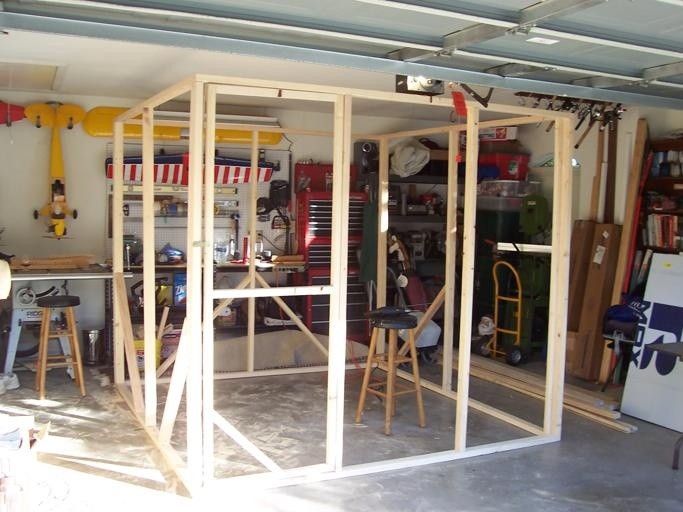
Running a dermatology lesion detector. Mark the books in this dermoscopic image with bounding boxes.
[625,148,683,296]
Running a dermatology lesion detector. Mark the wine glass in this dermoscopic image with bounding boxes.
[19,255,33,272]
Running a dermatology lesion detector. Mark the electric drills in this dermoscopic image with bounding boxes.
[363,306,422,317]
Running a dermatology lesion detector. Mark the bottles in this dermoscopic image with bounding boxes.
[214,301,256,327]
[155,253,169,264]
[215,234,236,263]
[404,183,418,210]
[259,147,264,161]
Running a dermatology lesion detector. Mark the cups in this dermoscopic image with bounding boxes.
[263,250,272,259]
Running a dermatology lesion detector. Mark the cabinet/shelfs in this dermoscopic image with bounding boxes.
[386,175,465,340]
[608,138,682,385]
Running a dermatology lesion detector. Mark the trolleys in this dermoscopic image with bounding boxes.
[474,260,524,369]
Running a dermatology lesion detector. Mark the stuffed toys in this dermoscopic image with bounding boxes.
[476,311,495,336]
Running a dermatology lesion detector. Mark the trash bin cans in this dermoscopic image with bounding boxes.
[82,329,105,366]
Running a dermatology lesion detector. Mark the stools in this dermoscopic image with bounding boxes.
[34,295,85,399]
[355,314,426,434]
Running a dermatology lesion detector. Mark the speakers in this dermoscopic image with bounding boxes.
[354,142,376,169]
